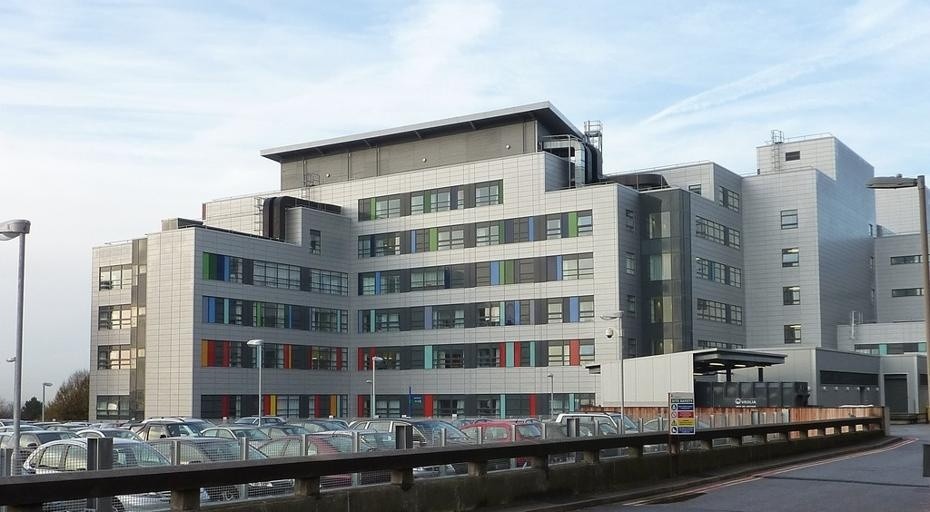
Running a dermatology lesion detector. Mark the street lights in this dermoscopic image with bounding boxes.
[865,172,930,414]
[0,219,31,476]
[600,310,624,419]
[41,382,52,422]
[366,356,383,420]
[547,373,554,417]
[247,338,265,426]
